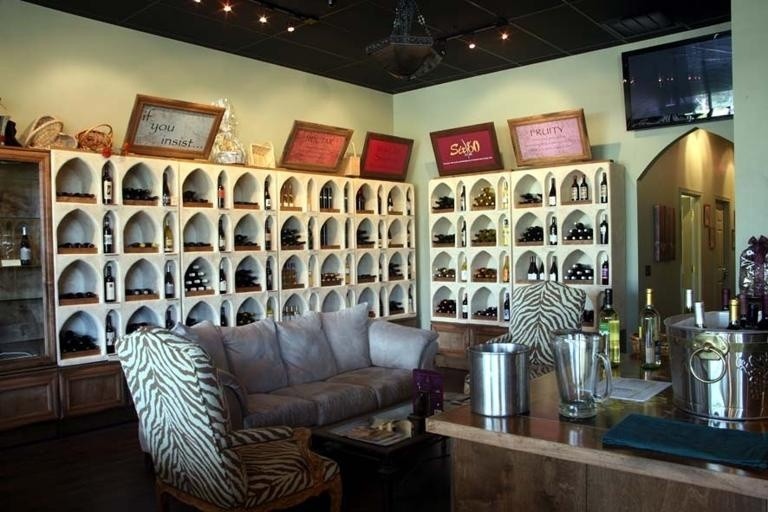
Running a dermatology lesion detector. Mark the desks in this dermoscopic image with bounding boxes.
[425,349,767,512]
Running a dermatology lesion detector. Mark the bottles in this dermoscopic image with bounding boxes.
[598,289,766,369]
[432,173,608,323]
[20,165,414,354]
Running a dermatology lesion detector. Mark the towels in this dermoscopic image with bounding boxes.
[601,412,768,470]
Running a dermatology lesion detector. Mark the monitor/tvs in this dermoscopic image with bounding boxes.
[621,29,733,131]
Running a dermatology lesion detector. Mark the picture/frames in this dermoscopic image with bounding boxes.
[121,94,226,159]
[360,130,415,182]
[507,108,592,167]
[278,119,354,172]
[429,120,504,177]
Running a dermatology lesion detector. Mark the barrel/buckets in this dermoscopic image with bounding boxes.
[664,311,768,421]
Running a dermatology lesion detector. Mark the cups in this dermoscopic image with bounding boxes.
[550,329,613,420]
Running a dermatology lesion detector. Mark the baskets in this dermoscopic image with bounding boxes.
[630,333,669,357]
[17,113,114,153]
[246,143,275,170]
[338,154,361,178]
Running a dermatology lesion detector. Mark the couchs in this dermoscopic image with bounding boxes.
[174,301,439,429]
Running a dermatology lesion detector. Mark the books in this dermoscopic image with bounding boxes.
[329,419,408,446]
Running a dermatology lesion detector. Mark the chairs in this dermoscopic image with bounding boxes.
[463,280,587,396]
[114,326,341,512]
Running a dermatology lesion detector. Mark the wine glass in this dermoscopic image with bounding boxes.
[0,234,15,260]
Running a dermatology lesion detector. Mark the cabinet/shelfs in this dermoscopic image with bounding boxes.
[426,172,512,368]
[0,147,58,428]
[180,162,279,333]
[278,171,357,319]
[512,162,626,328]
[50,146,184,416]
[355,181,418,321]
